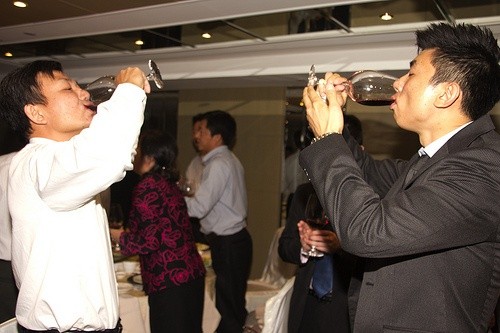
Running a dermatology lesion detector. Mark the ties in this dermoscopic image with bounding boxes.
[402,154,431,189]
[312,218,335,297]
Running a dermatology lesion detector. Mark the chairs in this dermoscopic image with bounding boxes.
[246,227,296,333]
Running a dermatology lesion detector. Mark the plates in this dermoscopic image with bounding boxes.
[112,273,142,295]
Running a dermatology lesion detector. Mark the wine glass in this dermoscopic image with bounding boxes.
[80,59,164,111]
[308,64,399,106]
[108,204,125,251]
[300,192,329,257]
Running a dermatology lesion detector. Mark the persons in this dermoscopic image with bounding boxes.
[299,21,500,333]
[0,109,365,333]
[0,60,150,333]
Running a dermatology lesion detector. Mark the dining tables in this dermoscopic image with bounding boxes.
[110,222,222,333]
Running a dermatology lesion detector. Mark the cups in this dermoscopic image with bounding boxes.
[181,177,195,197]
[123,262,136,273]
